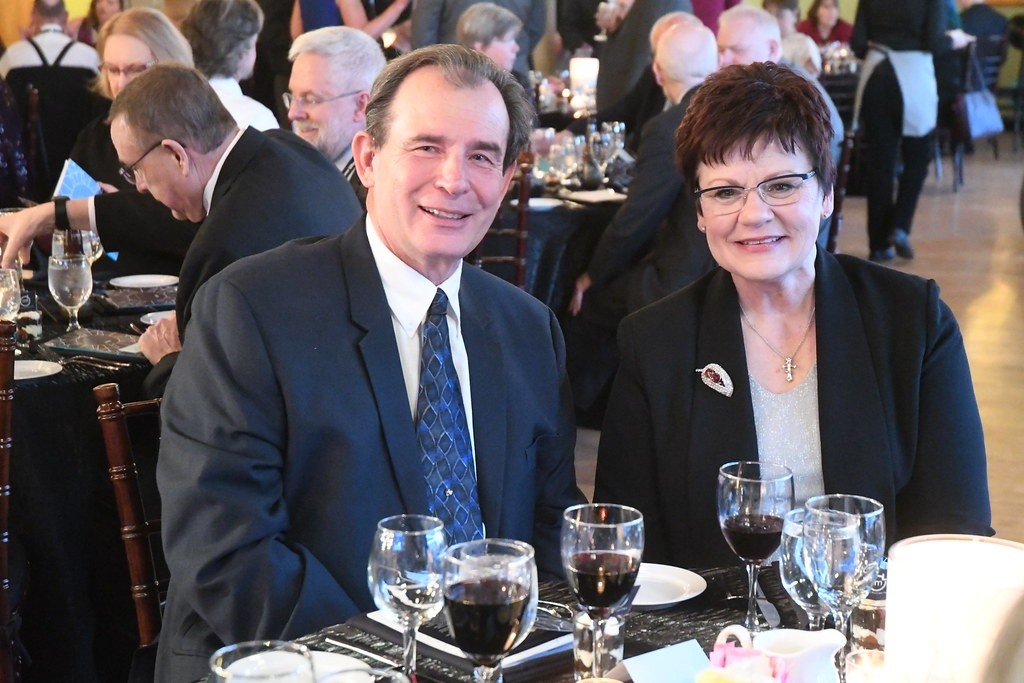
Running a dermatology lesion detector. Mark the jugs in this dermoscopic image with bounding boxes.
[711,625,847,682]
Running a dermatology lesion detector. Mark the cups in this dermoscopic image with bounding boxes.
[52,229,94,268]
[315,668,411,683]
[0,268,21,321]
[849,555,887,654]
[205,640,316,683]
[573,610,626,683]
[529,121,625,191]
[845,651,887,683]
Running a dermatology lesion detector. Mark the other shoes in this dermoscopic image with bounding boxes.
[886,226,913,258]
[867,248,895,261]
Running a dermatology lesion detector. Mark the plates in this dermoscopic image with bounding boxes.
[311,651,375,683]
[631,563,708,611]
[510,198,564,212]
[109,275,179,287]
[13,359,63,380]
[140,310,175,324]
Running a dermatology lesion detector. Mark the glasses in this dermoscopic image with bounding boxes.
[692,168,820,215]
[96,60,155,74]
[117,139,187,183]
[280,89,363,107]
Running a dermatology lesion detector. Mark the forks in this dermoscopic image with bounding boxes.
[37,343,131,372]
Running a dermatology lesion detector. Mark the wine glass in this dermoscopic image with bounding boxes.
[48,255,94,332]
[366,514,448,683]
[594,2,615,41]
[779,508,860,632]
[718,461,796,635]
[802,495,886,683]
[441,539,539,683]
[559,503,645,683]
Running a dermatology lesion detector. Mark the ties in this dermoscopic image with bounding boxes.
[411,286,484,557]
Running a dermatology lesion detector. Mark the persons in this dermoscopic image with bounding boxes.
[588,59,992,572]
[0,0,547,216]
[568,21,721,313]
[0,65,365,413]
[553,0,1024,261]
[73,6,203,275]
[152,42,592,683]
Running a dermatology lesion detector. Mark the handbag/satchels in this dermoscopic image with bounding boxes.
[957,43,1006,140]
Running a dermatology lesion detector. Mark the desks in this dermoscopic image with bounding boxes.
[195,552,891,683]
[13,263,184,683]
[474,188,631,311]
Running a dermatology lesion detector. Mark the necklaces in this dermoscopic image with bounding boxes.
[739,304,815,382]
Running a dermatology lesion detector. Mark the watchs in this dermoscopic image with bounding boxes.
[52,195,71,231]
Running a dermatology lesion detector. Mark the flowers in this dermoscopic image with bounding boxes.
[699,642,794,683]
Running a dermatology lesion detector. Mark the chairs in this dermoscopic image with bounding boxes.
[1,34,1015,683]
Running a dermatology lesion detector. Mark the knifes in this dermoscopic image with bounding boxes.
[754,580,780,628]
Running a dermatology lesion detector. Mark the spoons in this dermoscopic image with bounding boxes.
[129,322,144,334]
